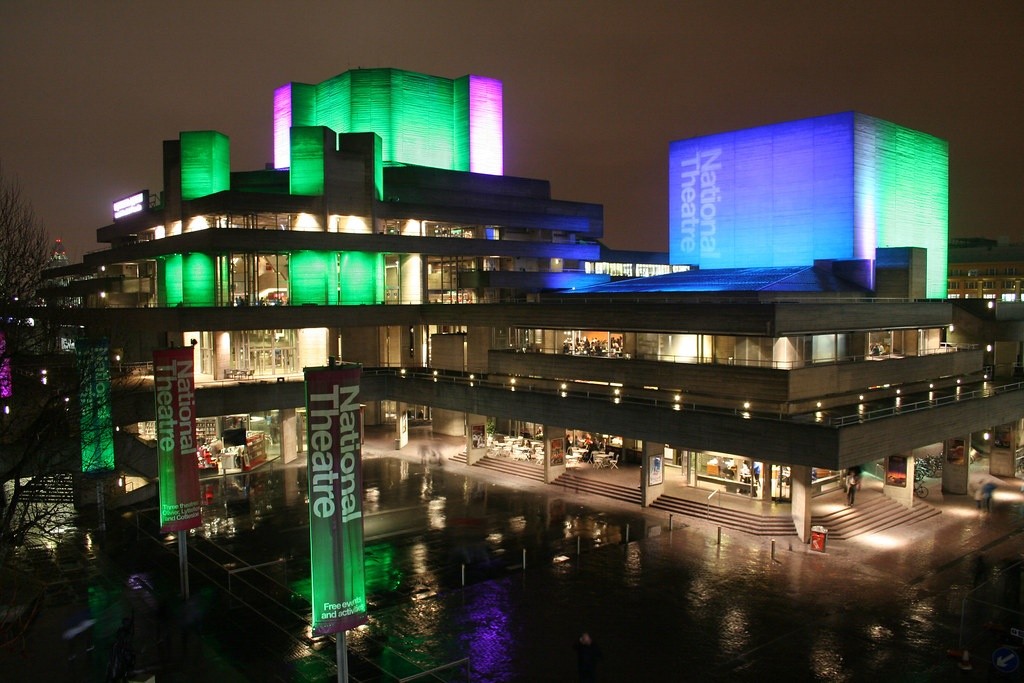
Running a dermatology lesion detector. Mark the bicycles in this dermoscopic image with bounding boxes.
[914,450,943,498]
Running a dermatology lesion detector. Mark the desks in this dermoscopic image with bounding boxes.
[531,442,540,450]
[494,444,509,449]
[571,446,588,462]
[593,454,612,468]
[517,448,532,461]
[566,455,582,464]
[504,439,519,444]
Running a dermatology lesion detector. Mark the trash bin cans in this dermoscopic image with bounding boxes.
[811,527,828,552]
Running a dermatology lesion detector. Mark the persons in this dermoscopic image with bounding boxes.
[973,479,998,513]
[869,342,885,357]
[845,472,861,507]
[236,295,269,305]
[526,440,532,454]
[564,336,623,356]
[565,434,607,463]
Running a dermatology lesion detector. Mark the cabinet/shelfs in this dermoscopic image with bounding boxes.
[218,431,265,475]
[138,415,250,473]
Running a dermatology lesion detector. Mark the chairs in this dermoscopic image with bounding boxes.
[231,370,242,380]
[471,434,588,468]
[593,455,602,470]
[239,370,247,379]
[225,369,233,379]
[590,451,598,464]
[611,454,619,468]
[248,369,255,380]
[607,452,614,466]
